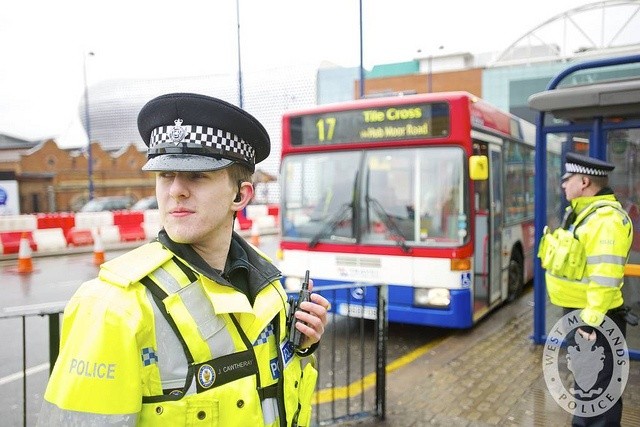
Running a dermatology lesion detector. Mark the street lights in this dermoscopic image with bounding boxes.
[83,51,96,200]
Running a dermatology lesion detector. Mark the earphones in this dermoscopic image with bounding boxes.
[234,193,241,203]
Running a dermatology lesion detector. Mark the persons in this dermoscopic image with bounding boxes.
[43,92,329,427]
[537,151,634,427]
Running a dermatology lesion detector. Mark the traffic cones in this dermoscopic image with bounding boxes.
[17,230,34,273]
[94,226,106,265]
[251,221,260,248]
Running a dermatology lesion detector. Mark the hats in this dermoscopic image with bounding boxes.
[560,152,615,181]
[137,93,270,174]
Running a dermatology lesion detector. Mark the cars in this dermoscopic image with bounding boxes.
[77,195,136,212]
[130,196,158,209]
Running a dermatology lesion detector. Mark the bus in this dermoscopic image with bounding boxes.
[278,91,564,331]
